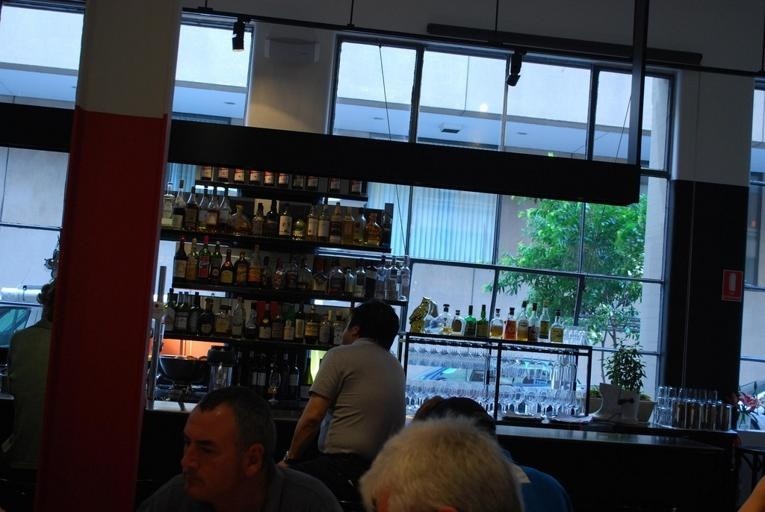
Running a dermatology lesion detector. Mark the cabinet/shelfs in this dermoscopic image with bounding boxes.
[146,166,409,417]
[399,331,592,418]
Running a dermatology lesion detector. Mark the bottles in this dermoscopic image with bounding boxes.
[164,168,408,412]
[430,300,563,344]
[655,384,737,434]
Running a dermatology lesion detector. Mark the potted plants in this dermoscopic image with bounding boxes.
[582,339,658,424]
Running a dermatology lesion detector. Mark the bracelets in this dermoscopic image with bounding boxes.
[283,450,298,466]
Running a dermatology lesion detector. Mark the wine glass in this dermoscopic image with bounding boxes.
[407,345,582,423]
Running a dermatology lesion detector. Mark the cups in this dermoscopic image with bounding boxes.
[562,325,586,345]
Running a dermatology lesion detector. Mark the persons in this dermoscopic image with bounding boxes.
[737,472,765,512]
[134,386,344,512]
[356,415,523,512]
[413,395,573,512]
[276,301,405,501]
[4,277,60,482]
